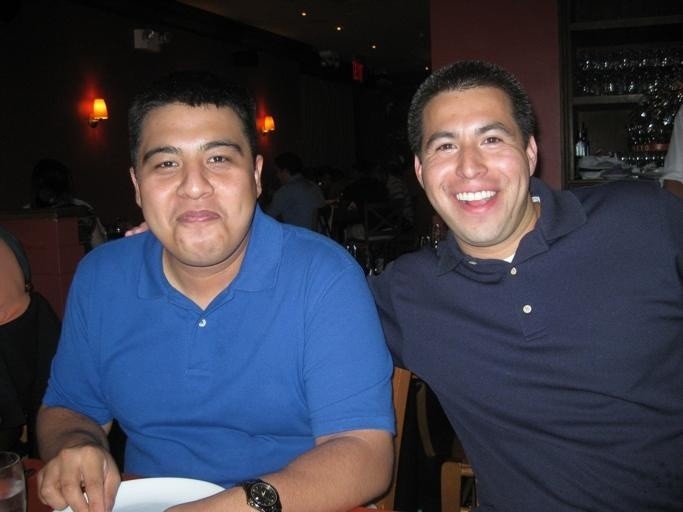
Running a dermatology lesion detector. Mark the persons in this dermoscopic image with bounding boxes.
[0,226,36,410]
[124,60,683,512]
[22,158,108,251]
[332,133,414,276]
[663,103,683,198]
[266,154,327,232]
[36,69,397,512]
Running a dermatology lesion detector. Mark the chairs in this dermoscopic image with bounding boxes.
[0,195,480,512]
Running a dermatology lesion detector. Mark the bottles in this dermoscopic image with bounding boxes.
[573,132,590,156]
[626,100,679,170]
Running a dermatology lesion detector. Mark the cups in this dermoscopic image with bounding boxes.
[0,451,25,512]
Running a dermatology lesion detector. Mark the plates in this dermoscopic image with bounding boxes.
[51,477,225,511]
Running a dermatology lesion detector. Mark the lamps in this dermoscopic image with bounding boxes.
[87,98,109,128]
[261,115,280,136]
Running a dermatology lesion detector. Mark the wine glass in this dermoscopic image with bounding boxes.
[575,47,680,94]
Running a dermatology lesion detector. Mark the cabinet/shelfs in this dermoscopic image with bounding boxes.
[568,12,683,189]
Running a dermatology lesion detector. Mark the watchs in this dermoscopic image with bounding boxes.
[235,478,282,512]
